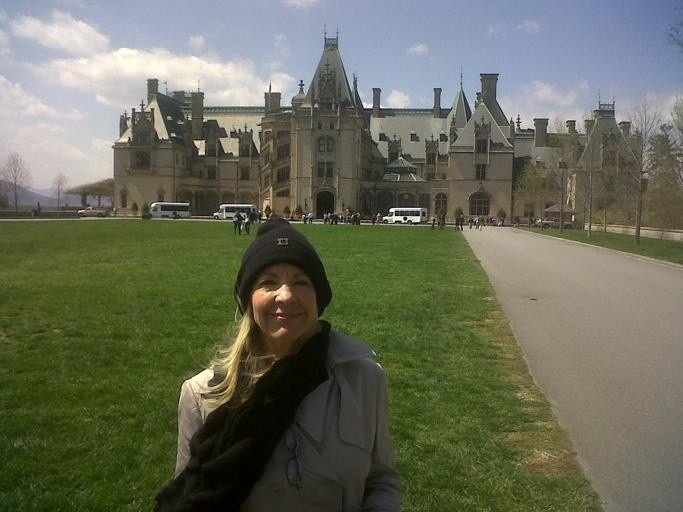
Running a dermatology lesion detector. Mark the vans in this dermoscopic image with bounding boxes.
[535,218,542,225]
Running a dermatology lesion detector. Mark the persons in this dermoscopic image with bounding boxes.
[233,212,543,236]
[173,216,403,511]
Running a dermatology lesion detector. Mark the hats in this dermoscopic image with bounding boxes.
[233,217,331,318]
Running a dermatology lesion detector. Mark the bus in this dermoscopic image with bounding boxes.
[148,202,190,219]
[213,203,256,220]
[382,207,427,225]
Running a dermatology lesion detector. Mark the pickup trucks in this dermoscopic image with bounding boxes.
[78,207,106,217]
[538,217,573,229]
